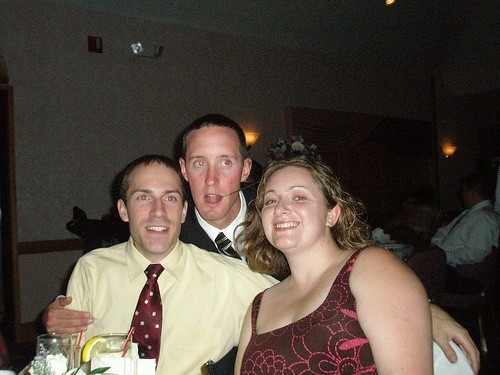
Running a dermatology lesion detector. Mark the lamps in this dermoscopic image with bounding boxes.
[441,146,457,160]
[244,132,262,151]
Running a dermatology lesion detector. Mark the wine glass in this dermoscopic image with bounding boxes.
[98,331,133,358]
[35,332,71,375]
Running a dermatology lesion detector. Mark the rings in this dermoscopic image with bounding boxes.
[49,331,57,338]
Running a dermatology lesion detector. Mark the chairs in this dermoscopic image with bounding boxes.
[438,246,500,353]
[403,246,449,307]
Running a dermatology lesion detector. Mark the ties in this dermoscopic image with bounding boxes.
[130,263,165,372]
[215,232,243,262]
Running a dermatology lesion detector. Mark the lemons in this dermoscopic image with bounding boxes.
[81,336,109,362]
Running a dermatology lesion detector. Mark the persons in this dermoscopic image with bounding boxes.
[53,154,481,375]
[375,184,447,263]
[472,145,500,206]
[430,170,500,296]
[234,157,434,375]
[34,113,293,375]
